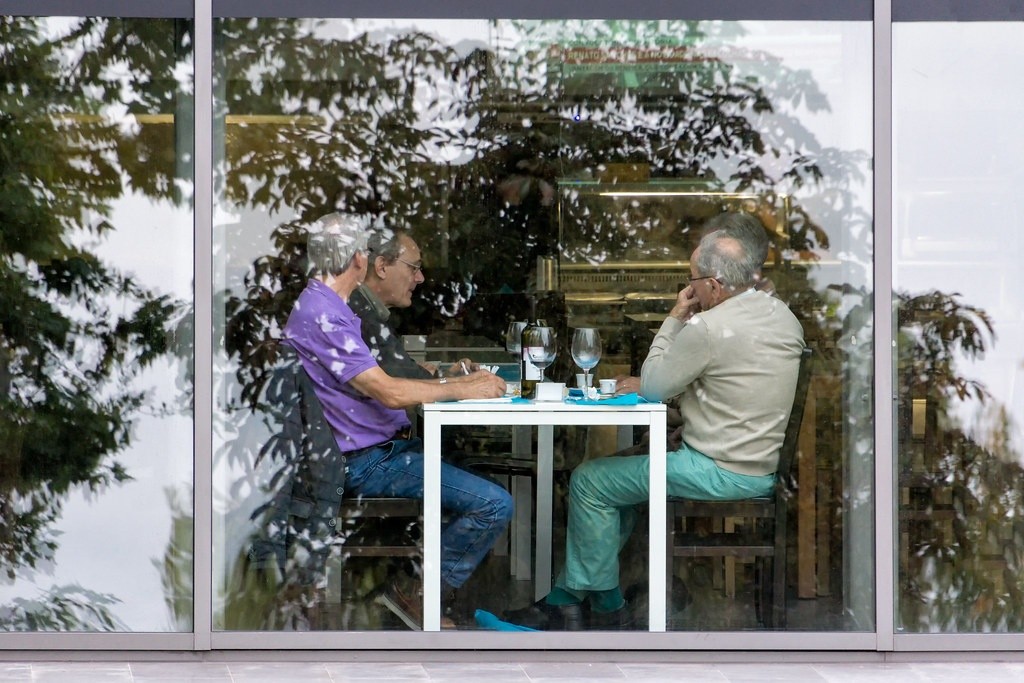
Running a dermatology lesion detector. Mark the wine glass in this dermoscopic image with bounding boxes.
[528,327,557,405]
[571,328,602,403]
[506,322,528,400]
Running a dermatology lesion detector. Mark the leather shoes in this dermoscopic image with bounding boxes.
[501,598,587,630]
[585,598,635,629]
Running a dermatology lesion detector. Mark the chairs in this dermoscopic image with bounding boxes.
[641,332,971,630]
[231,308,447,631]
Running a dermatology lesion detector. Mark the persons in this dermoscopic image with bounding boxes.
[281,213,514,631]
[505,212,804,632]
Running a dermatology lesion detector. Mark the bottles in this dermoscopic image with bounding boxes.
[521,308,544,400]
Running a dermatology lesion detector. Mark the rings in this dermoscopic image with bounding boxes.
[624,385,625,387]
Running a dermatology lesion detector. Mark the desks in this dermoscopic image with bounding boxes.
[400,334,667,634]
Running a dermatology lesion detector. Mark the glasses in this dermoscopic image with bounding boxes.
[354,246,375,260]
[382,252,422,275]
[686,273,724,288]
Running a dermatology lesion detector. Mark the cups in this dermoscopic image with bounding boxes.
[599,379,618,395]
[576,374,594,389]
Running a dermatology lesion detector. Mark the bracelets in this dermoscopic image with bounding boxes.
[440,377,446,384]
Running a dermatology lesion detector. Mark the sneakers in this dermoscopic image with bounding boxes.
[380,572,456,633]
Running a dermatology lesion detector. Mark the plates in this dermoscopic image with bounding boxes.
[601,395,614,399]
